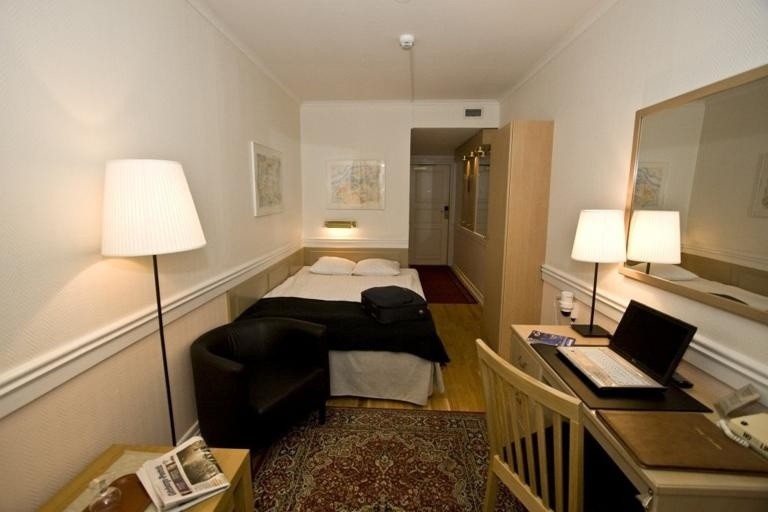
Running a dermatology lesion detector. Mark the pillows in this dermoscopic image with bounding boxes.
[311,256,356,275]
[351,258,401,276]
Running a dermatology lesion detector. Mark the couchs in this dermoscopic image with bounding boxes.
[191,316,332,461]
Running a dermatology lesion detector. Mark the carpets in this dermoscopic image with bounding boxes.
[409,264,477,304]
[236,404,529,512]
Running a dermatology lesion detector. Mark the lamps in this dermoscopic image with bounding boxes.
[476,146,486,158]
[325,221,357,230]
[462,154,468,161]
[101,159,208,447]
[572,209,626,339]
[627,209,682,276]
[468,149,476,159]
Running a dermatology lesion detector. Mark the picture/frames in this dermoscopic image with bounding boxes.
[251,141,286,217]
[325,160,386,211]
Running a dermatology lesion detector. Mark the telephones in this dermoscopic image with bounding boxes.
[717,412,767,461]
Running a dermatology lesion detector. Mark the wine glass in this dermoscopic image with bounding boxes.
[88,472,123,511]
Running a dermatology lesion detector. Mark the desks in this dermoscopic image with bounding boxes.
[30,445,258,512]
[508,319,768,512]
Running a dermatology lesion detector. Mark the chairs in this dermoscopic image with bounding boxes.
[473,336,587,512]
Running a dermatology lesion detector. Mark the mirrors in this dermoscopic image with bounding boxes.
[615,64,767,332]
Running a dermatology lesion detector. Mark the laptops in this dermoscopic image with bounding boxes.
[557,300,697,395]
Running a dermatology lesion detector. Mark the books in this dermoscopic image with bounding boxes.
[595,408,768,477]
[527,329,576,348]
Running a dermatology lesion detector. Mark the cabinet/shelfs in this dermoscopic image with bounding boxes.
[481,121,553,354]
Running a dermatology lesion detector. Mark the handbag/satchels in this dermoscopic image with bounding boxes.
[361,285,432,328]
